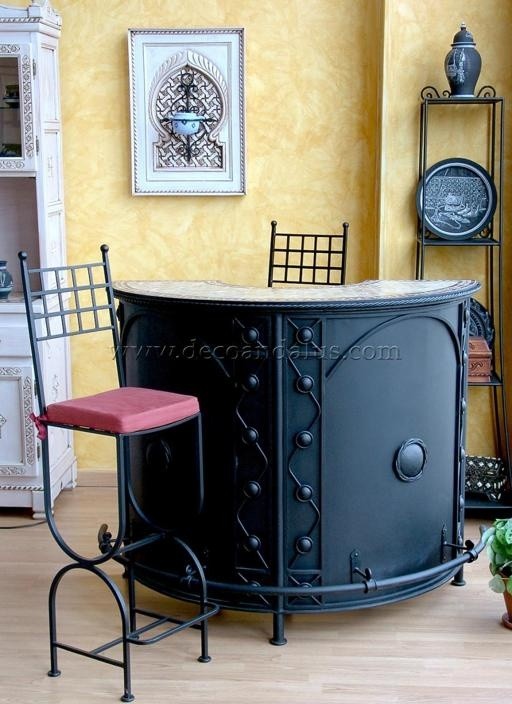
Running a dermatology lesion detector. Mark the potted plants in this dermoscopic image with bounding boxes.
[484,517,511,628]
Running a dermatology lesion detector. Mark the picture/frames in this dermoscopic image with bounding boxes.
[127,25,248,198]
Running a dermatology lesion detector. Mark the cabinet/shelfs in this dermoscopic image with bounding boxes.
[414,84,512,512]
[0,0,64,181]
[0,289,80,520]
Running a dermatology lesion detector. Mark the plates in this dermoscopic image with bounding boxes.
[469,297,496,351]
[414,158,497,242]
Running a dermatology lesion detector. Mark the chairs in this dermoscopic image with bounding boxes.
[267,218,350,287]
[17,242,221,702]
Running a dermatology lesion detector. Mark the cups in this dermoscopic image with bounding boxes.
[172,111,199,136]
